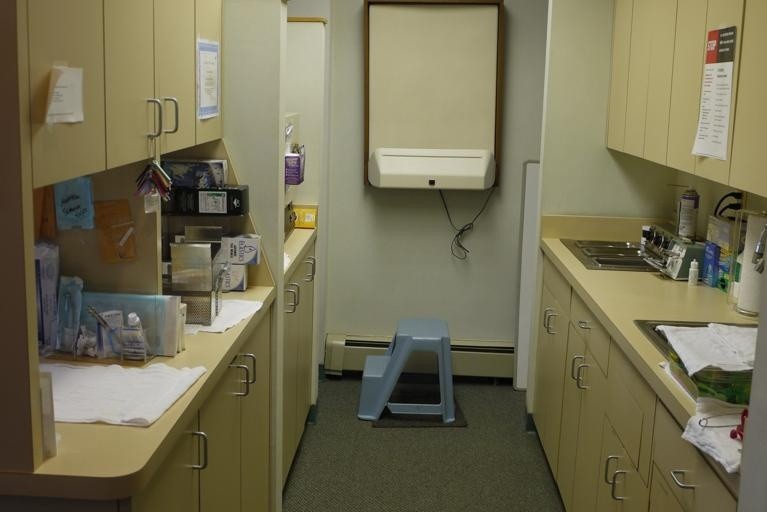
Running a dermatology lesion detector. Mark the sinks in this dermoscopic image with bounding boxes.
[560,236,641,248]
[583,258,653,272]
[572,248,641,256]
[634,318,758,414]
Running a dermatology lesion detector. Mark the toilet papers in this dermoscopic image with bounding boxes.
[737,213,767,313]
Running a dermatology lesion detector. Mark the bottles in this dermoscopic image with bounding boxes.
[127,312,144,337]
[687,258,700,287]
[676,183,701,239]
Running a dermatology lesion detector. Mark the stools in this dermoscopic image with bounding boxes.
[356,318,456,425]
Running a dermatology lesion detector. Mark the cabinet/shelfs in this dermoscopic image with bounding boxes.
[531,251,739,512]
[283,239,317,491]
[606,1,767,199]
[199,305,272,512]
[28,0,224,191]
[0,410,208,512]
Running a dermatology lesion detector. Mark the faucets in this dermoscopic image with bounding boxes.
[755,226,766,258]
